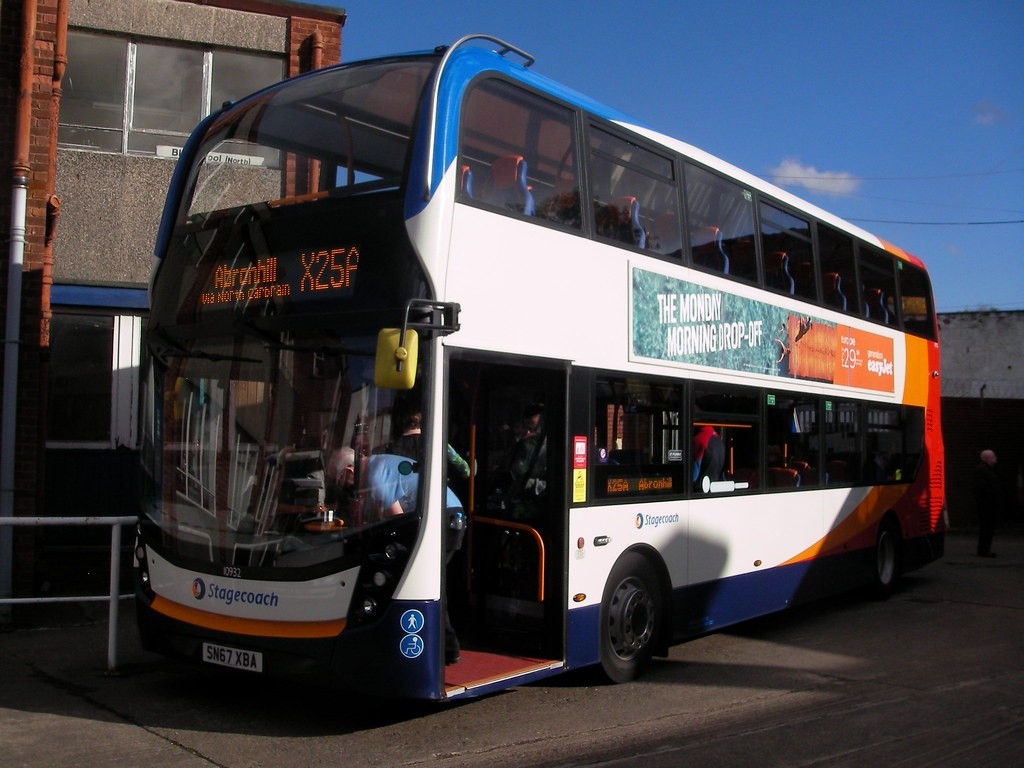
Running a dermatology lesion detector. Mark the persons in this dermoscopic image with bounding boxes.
[689,417,728,498]
[384,408,482,491]
[487,398,554,578]
[326,448,465,665]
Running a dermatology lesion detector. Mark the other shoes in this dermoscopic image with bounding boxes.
[976,551,997,558]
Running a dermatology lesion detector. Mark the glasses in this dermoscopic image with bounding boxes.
[343,464,354,491]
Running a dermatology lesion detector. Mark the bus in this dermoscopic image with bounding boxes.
[128,34,944,707]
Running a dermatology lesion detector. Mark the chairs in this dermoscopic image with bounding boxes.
[649,213,679,260]
[823,272,847,311]
[727,240,753,279]
[595,195,646,249]
[457,165,473,200]
[692,226,730,274]
[764,250,795,295]
[869,288,888,323]
[477,155,533,217]
[828,460,847,482]
[770,467,802,492]
[797,260,815,299]
[850,285,869,317]
